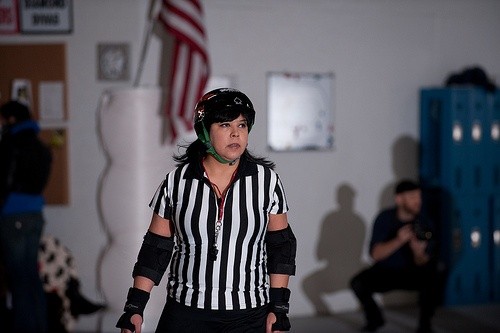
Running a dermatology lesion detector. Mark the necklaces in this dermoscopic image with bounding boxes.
[208,166,237,260]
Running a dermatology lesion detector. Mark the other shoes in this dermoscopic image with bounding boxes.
[70,298,106,321]
[361,317,384,333]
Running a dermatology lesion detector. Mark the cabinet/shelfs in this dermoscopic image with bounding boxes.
[418,87,500,305]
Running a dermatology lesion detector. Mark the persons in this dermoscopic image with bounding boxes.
[0,99,53,332]
[115,88,297,333]
[351,180,443,333]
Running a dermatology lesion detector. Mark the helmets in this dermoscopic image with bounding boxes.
[194,88,255,135]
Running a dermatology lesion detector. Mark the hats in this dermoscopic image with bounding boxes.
[393,178,419,195]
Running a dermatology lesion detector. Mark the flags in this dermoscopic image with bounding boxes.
[151,0,212,138]
